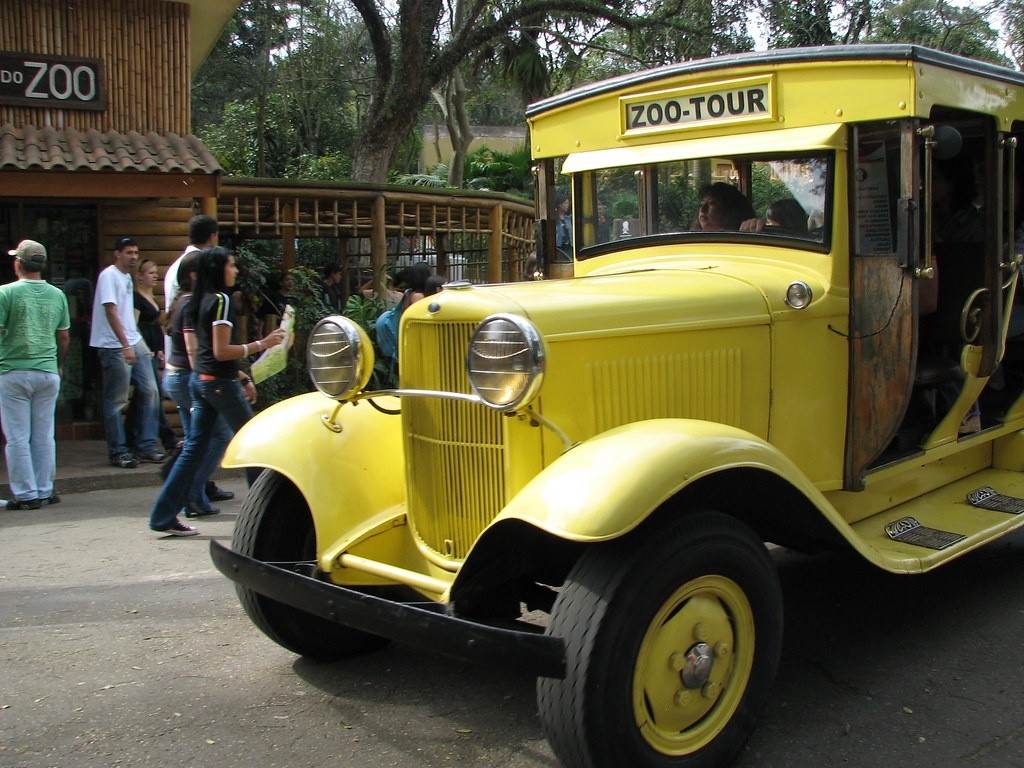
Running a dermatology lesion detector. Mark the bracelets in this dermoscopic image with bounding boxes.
[255,340,262,352]
[122,347,131,350]
[240,376,252,387]
[242,344,248,358]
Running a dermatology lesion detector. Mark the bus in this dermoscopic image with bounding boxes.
[210,41,1024,768]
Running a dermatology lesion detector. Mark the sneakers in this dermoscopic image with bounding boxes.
[165,521,198,536]
[111,453,139,468]
[135,450,171,463]
[958,416,981,433]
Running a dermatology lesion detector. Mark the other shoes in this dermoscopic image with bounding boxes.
[184,503,220,517]
[40,494,60,506]
[209,488,234,501]
[0,500,8,507]
[169,441,185,456]
[7,498,40,510]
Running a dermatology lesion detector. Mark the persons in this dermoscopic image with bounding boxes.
[918,155,1024,437]
[231,261,448,391]
[556,195,611,259]
[0,240,70,509]
[90,216,286,537]
[697,183,824,235]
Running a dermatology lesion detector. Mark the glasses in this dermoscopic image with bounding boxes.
[121,238,131,243]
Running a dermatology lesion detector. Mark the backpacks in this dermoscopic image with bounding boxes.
[376,288,411,361]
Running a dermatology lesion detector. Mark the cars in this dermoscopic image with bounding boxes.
[355,236,470,287]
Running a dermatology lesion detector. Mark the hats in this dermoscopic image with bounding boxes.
[7,240,47,264]
